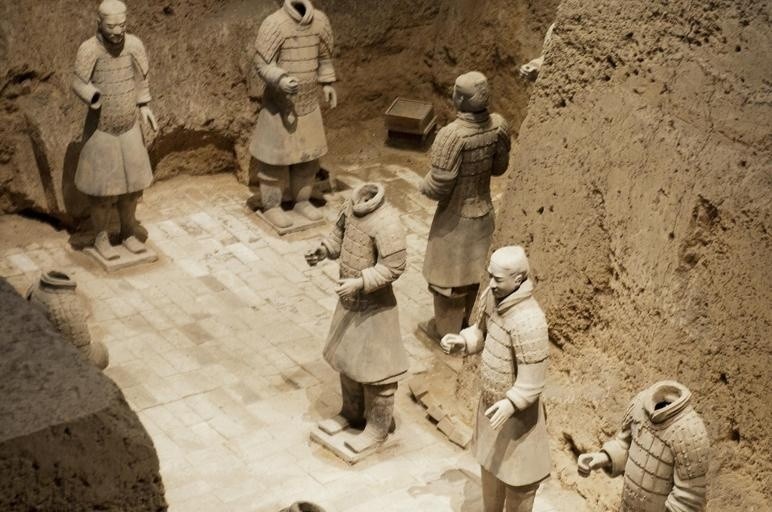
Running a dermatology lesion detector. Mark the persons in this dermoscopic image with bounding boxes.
[24,270,109,370]
[440,246,550,512]
[305,183,409,453]
[248,0,337,227]
[71,0,158,260]
[418,71,511,340]
[577,381,711,511]
